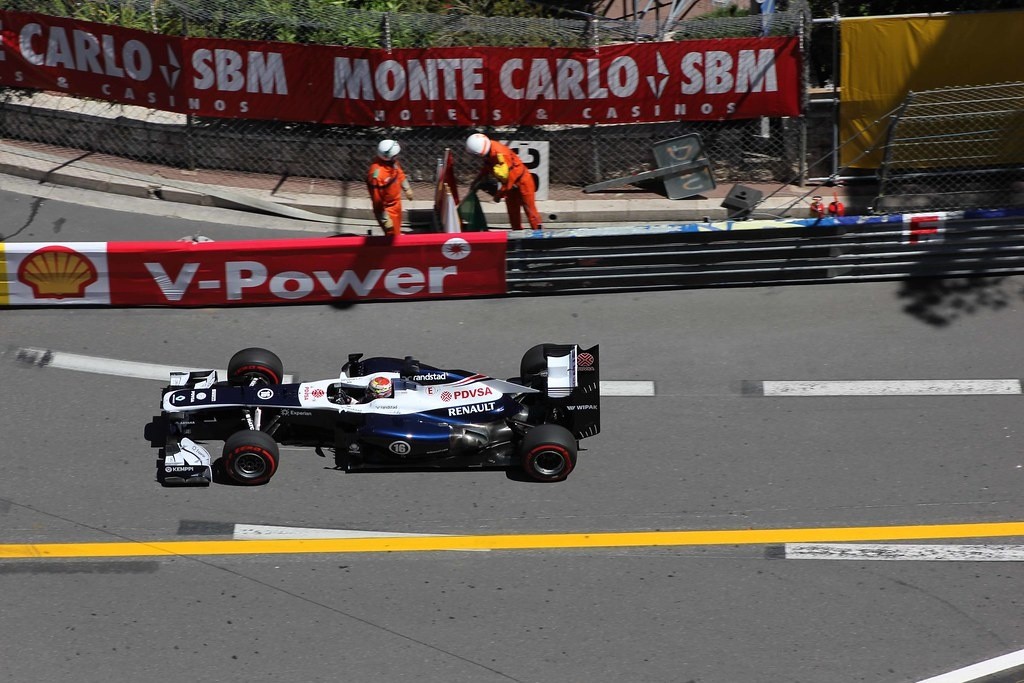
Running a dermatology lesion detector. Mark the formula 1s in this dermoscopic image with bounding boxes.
[160,340,600,488]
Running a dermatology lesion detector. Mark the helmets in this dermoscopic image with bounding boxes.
[464,133,491,158]
[377,139,401,161]
[368,377,392,398]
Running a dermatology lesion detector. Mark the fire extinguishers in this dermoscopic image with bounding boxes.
[828,192,846,217]
[807,196,825,220]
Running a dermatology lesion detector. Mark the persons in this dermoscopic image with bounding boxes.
[366,139,413,237]
[466,134,544,230]
[339,377,392,407]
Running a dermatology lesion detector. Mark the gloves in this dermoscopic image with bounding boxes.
[375,210,394,231]
[400,178,414,201]
[346,395,359,404]
